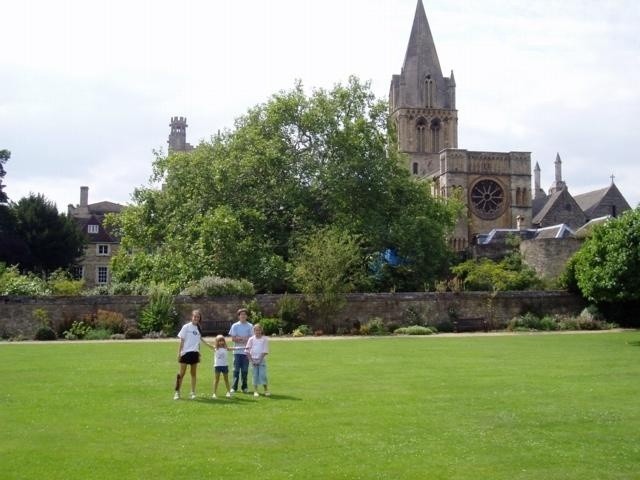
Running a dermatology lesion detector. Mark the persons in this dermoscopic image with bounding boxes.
[201,335,249,400]
[229,309,253,394]
[174,310,202,401]
[244,324,271,397]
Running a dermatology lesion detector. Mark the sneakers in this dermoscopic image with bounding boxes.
[253,392,259,397]
[225,392,230,397]
[211,393,216,398]
[230,388,234,392]
[189,391,197,400]
[173,391,180,400]
[264,391,270,396]
[242,388,248,393]
